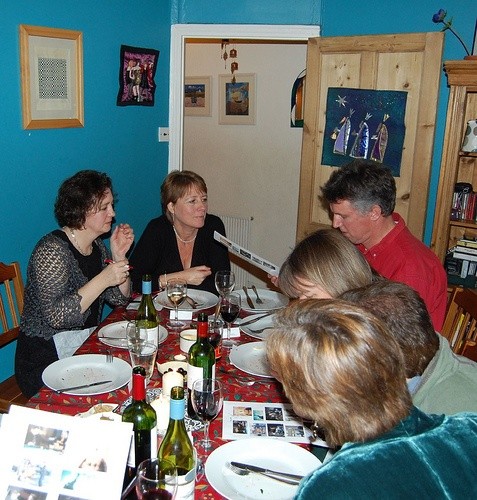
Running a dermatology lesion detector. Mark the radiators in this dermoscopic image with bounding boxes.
[220,214,254,289]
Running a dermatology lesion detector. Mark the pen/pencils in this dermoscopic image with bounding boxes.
[104,258,135,270]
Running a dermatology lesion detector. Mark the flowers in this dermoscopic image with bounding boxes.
[432,9,472,55]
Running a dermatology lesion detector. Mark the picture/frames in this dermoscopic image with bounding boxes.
[19,24,84,129]
[117,45,159,106]
[217,73,259,126]
[183,76,213,118]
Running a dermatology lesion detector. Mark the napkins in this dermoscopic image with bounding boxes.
[53,326,97,359]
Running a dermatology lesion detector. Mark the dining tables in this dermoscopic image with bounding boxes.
[28,293,288,500]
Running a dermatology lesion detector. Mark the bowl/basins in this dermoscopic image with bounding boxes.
[180,329,197,353]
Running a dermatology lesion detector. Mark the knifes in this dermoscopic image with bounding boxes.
[57,380,113,393]
[239,312,274,326]
[98,336,126,340]
[230,461,304,481]
[242,286,255,309]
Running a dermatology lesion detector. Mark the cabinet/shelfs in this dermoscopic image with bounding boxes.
[429,60,477,292]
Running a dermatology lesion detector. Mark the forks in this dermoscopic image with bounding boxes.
[235,378,277,386]
[225,462,299,486]
[247,327,275,334]
[252,285,264,304]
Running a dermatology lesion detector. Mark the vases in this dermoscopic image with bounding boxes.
[463,54,477,60]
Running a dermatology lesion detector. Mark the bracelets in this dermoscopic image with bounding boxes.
[160,274,167,288]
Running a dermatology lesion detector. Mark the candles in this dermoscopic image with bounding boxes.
[162,371,183,395]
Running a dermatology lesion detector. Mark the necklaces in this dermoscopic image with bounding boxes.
[70,229,92,255]
[173,225,197,243]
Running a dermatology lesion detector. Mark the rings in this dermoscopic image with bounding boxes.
[125,272,127,277]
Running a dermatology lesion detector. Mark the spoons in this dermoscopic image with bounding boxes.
[178,287,204,306]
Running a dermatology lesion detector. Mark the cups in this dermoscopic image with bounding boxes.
[461,119,477,152]
[135,458,178,500]
[126,320,159,385]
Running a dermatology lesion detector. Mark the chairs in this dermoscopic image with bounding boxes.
[0,262,31,413]
[441,284,477,363]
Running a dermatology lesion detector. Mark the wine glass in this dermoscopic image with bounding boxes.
[165,278,187,329]
[191,377,224,458]
[219,291,241,348]
[215,271,236,321]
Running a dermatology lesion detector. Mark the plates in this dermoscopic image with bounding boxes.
[42,353,133,396]
[231,288,289,377]
[204,436,322,500]
[156,288,220,311]
[98,320,168,350]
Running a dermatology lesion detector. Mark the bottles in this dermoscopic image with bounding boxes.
[187,311,216,421]
[136,274,159,365]
[121,366,197,500]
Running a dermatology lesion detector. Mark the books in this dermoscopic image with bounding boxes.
[451,192,477,220]
[453,239,477,346]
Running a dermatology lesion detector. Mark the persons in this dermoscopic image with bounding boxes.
[341,281,477,414]
[277,229,384,300]
[129,170,231,297]
[14,169,135,399]
[271,160,447,332]
[265,299,477,500]
